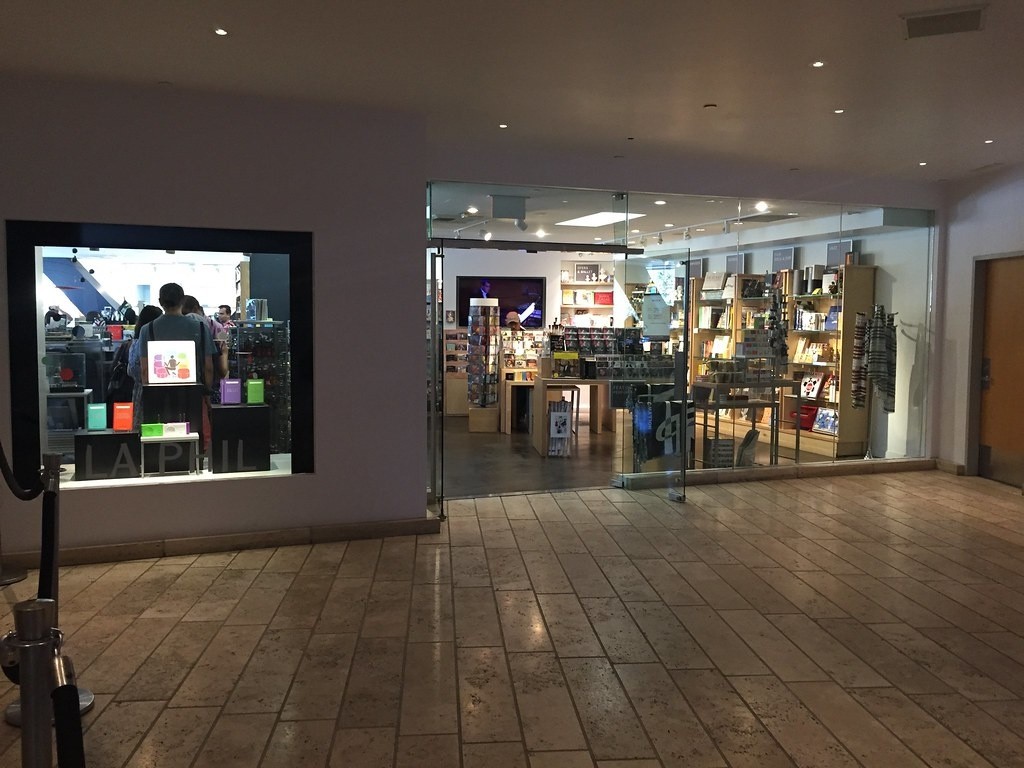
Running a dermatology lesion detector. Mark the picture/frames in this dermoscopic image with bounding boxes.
[690,258,703,278]
[771,246,795,274]
[575,263,601,282]
[725,253,744,275]
[827,240,853,269]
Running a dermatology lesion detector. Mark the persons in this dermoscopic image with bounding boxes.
[218,305,236,349]
[477,279,491,298]
[182,295,229,404]
[127,305,163,433]
[506,311,525,331]
[138,283,218,457]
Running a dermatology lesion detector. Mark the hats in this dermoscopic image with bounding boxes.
[506,311,519,324]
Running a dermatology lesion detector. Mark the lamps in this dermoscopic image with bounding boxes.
[516,219,528,232]
[683,228,692,240]
[657,233,664,246]
[477,229,492,241]
[722,221,730,234]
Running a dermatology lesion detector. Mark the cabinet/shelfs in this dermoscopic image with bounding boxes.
[446,265,878,456]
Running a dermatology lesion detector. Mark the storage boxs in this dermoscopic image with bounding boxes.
[208,403,272,473]
[139,431,200,478]
[74,429,139,477]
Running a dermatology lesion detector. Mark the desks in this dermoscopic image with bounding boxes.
[45,320,121,455]
[690,380,801,470]
[691,399,780,469]
[532,374,648,457]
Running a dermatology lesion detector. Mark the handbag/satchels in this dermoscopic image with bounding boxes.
[107,339,133,402]
